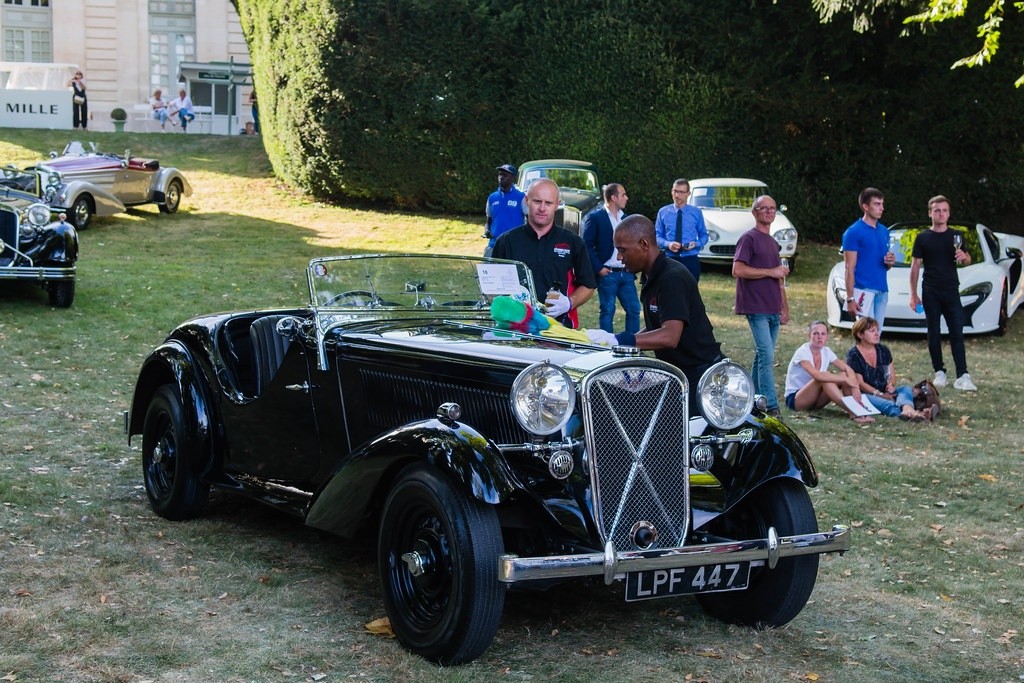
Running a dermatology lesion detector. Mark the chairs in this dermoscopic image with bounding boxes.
[132,104,212,135]
[251,315,308,397]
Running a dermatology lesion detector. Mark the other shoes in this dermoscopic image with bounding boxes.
[899,407,931,423]
[188,116,192,123]
[160,130,166,134]
[172,120,178,126]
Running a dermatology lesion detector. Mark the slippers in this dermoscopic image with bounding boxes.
[851,415,875,423]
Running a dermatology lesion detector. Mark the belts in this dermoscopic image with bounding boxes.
[603,265,627,272]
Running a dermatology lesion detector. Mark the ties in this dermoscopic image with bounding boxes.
[673,209,683,262]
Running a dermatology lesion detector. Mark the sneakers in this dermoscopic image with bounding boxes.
[954,373,978,390]
[933,371,946,387]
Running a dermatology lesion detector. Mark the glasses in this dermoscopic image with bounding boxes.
[754,206,776,212]
[672,188,688,194]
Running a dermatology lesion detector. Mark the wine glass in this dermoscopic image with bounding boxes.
[954,235,962,266]
[883,366,890,386]
[779,257,790,289]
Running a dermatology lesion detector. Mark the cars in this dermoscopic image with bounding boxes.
[0,141,194,232]
[124,252,850,668]
[0,166,82,309]
[518,159,600,236]
[685,178,798,277]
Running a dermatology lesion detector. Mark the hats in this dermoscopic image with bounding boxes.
[496,164,517,177]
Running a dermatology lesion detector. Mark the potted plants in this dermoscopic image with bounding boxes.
[110,109,127,131]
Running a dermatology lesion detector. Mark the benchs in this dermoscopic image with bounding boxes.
[97,153,160,171]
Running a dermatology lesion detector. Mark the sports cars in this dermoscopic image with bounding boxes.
[827,221,1024,336]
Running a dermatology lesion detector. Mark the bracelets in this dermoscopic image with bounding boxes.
[880,393,883,397]
[873,389,878,395]
[568,296,572,312]
[846,297,856,302]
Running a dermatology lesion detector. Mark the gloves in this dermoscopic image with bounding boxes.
[544,290,572,320]
[585,328,636,348]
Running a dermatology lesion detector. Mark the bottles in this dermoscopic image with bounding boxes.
[544,279,562,308]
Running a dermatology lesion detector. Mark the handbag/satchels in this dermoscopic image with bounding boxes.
[910,379,942,417]
[74,95,85,105]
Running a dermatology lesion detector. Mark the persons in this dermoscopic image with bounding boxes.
[249,90,261,135]
[732,195,790,423]
[582,183,641,334]
[67,71,89,131]
[483,163,528,264]
[587,214,727,417]
[910,195,977,391]
[150,89,177,133]
[842,187,896,340]
[169,90,195,133]
[846,316,938,421]
[784,321,876,423]
[655,178,709,283]
[489,178,598,329]
